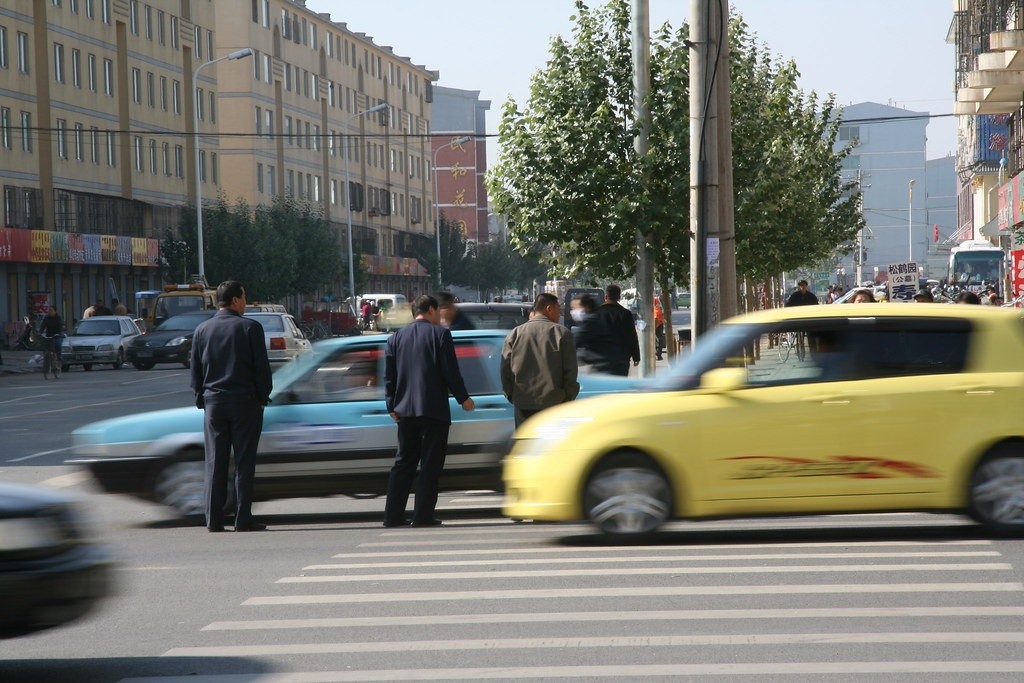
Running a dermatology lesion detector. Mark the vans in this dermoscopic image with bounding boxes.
[151,284,218,328]
[356,294,407,321]
[243,303,288,314]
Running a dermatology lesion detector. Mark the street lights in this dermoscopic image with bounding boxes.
[433,135,473,287]
[192,46,255,283]
[342,103,389,308]
[909,178,917,260]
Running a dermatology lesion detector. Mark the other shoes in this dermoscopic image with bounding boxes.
[234,523,267,532]
[656,355,663,361]
[208,526,224,532]
[56,363,61,370]
[413,519,442,528]
[384,519,412,527]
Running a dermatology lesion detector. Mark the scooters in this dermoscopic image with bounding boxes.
[13,316,49,352]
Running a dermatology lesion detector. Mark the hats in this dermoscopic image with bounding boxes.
[913,289,933,301]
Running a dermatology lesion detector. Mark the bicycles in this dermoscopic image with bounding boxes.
[778,331,805,363]
[36,333,62,381]
[300,317,330,341]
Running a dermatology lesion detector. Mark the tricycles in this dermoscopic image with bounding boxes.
[300,298,363,336]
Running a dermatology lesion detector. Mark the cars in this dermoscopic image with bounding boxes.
[499,302,1023,542]
[62,328,650,525]
[125,310,219,372]
[59,315,143,373]
[619,288,692,340]
[452,302,535,331]
[1,465,118,642]
[242,312,311,363]
[832,286,887,305]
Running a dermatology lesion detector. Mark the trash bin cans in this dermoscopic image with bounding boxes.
[677,329,691,355]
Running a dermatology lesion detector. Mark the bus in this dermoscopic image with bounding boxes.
[948,239,1005,304]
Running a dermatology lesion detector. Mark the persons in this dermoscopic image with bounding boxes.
[571,283,639,376]
[111,298,128,315]
[785,280,818,361]
[433,292,475,331]
[383,296,475,526]
[38,305,64,372]
[501,291,580,522]
[654,296,664,360]
[189,279,272,531]
[361,299,379,329]
[823,283,1004,306]
[83,304,97,319]
[94,300,113,316]
